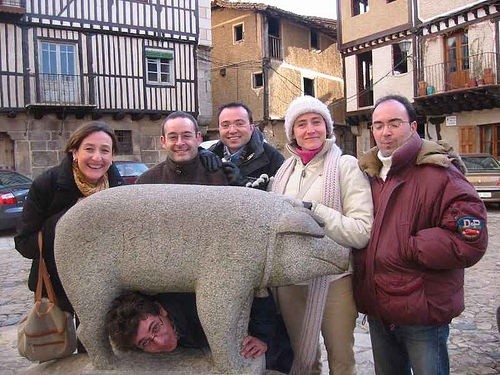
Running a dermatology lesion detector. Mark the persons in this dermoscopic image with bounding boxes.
[105,289,295,375]
[134,110,232,186]
[205,102,284,191]
[352,95,488,375]
[14,121,130,354]
[264,96,375,375]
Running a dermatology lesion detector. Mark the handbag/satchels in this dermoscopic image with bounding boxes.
[16,231,76,362]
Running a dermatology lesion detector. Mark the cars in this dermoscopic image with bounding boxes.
[113,161,149,184]
[0,169,33,231]
[459,152,500,204]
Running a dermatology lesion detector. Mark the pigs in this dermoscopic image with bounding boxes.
[58,184,349,375]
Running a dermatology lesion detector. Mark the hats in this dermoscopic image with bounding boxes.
[285,95,334,142]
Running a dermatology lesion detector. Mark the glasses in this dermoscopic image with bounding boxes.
[138,316,164,349]
[370,120,409,132]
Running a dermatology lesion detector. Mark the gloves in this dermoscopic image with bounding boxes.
[224,162,240,182]
[246,174,274,192]
[200,149,222,172]
